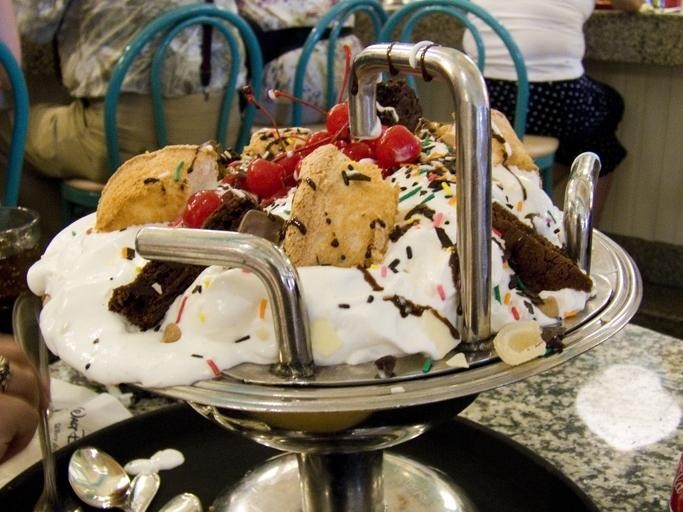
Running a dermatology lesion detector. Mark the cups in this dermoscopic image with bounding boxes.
[0,200,43,339]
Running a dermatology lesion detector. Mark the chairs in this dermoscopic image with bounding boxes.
[0,37,30,207]
[242,1,388,136]
[60,3,263,227]
[374,0,561,198]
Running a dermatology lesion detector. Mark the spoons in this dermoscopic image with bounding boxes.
[64,438,134,512]
[10,291,70,510]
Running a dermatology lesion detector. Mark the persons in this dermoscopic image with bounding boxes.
[238,0,362,127]
[1,0,247,183]
[0,332,47,465]
[460,0,643,234]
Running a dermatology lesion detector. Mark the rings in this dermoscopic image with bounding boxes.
[0,355,11,392]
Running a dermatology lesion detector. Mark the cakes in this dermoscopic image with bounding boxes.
[23,81,596,384]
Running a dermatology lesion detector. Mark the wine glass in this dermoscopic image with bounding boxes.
[37,227,647,511]
